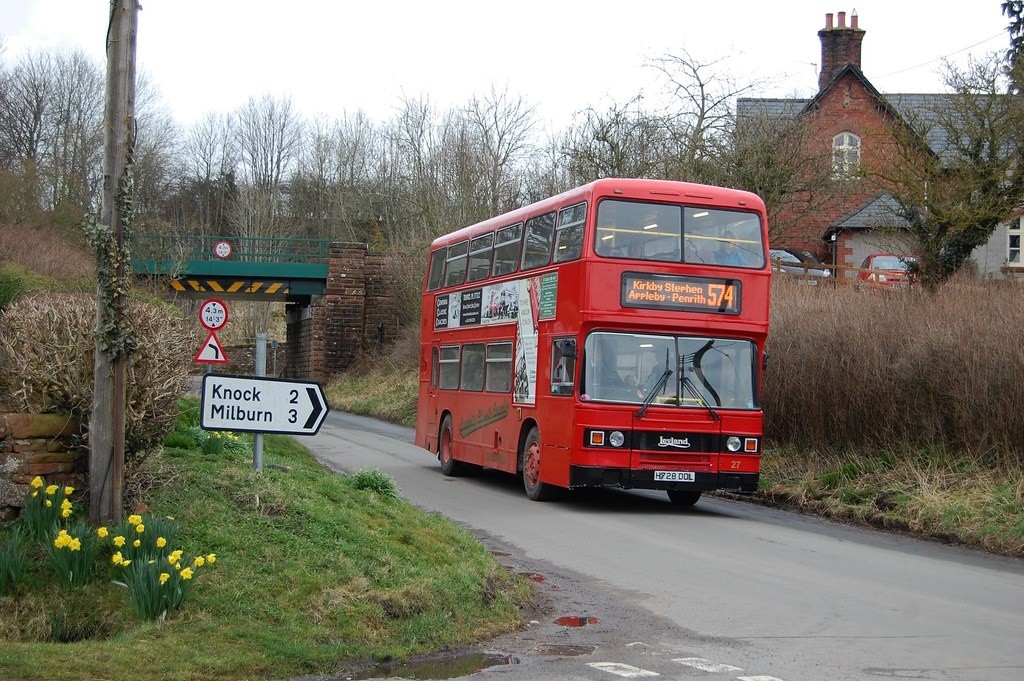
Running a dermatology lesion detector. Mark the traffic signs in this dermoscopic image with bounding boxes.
[202,374,330,436]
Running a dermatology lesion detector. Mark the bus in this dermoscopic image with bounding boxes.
[416,179,772,503]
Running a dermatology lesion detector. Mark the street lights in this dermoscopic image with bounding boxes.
[831,233,841,289]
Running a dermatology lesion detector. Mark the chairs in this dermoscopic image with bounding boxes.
[437,244,648,291]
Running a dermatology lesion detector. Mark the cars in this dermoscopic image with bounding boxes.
[855,255,923,288]
[769,247,832,278]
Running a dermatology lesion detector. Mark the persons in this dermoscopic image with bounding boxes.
[591,346,629,390]
[439,347,460,387]
[712,230,748,265]
[490,360,511,391]
[641,344,679,395]
[630,375,645,398]
[683,228,717,264]
[463,353,484,389]
